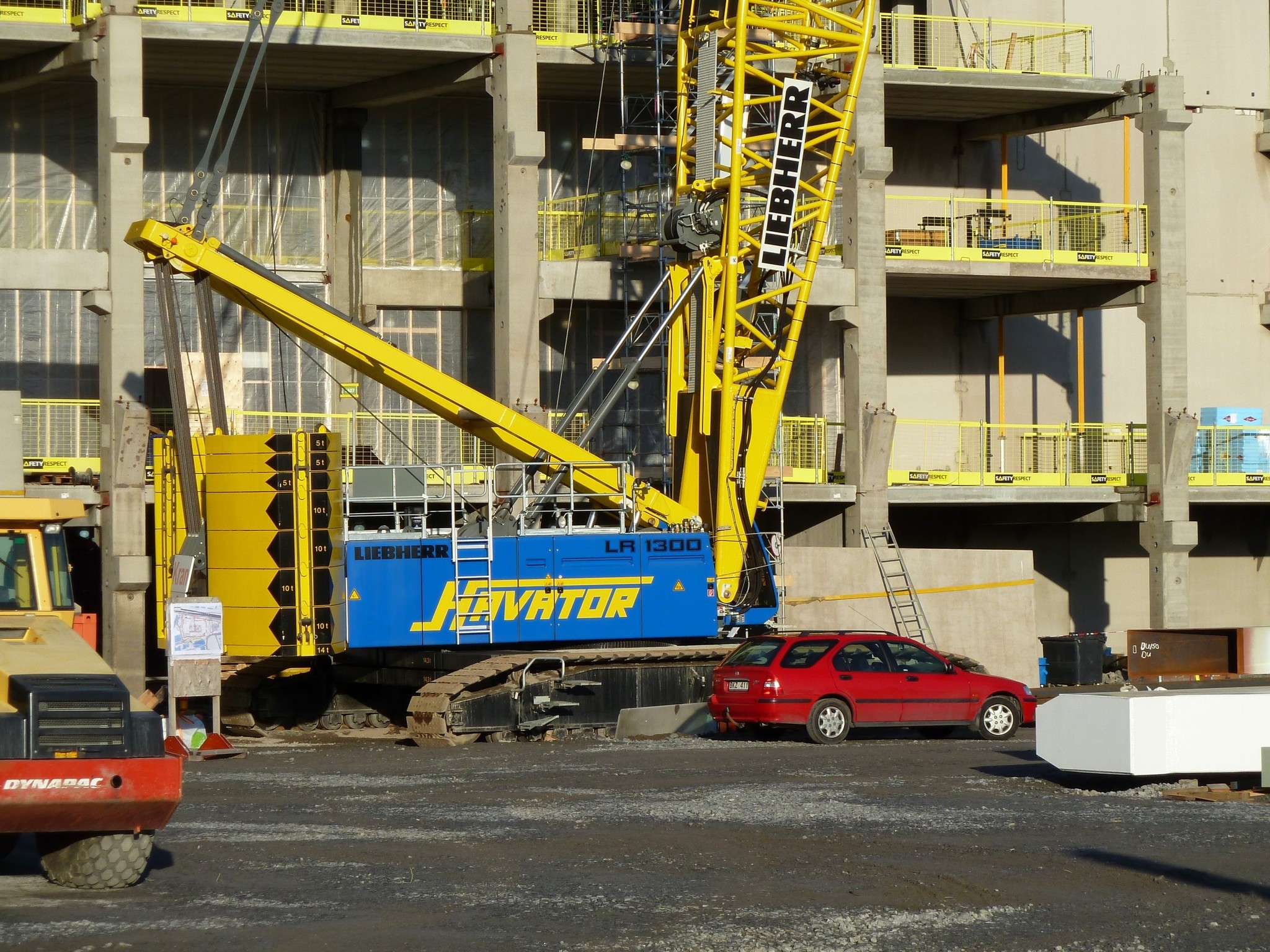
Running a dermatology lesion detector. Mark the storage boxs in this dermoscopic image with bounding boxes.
[1190,407,1270,472]
[980,235,1041,249]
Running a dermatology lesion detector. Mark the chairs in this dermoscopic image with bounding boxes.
[849,656,869,671]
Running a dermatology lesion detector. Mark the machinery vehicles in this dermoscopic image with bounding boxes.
[2,0,881,738]
[1,492,199,880]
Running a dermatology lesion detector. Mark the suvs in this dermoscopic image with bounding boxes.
[705,628,1038,746]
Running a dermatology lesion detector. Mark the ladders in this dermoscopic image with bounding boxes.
[864,521,938,664]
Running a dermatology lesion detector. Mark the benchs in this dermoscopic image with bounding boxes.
[805,653,846,672]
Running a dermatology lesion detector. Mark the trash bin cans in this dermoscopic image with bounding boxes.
[1040,633,1107,685]
